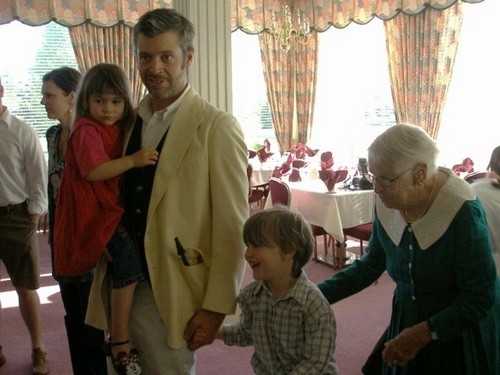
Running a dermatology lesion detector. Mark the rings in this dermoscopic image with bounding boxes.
[392,360,399,364]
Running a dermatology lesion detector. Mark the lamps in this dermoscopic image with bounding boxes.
[246,0,311,54]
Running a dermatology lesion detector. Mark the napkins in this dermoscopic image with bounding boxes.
[271,142,320,182]
[248,138,275,162]
[318,151,349,191]
[452,158,476,178]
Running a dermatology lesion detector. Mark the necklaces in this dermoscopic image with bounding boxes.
[402,175,438,224]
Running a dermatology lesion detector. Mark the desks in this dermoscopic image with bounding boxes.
[252,161,281,190]
[284,179,376,271]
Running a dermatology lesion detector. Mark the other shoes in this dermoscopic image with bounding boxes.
[105,334,143,375]
[30,344,51,375]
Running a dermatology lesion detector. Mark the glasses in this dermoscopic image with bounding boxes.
[365,167,417,187]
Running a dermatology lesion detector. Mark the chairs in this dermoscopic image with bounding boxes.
[339,222,373,267]
[246,164,265,216]
[268,177,328,263]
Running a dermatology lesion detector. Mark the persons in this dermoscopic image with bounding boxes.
[51,64,159,375]
[0,81,49,375]
[40,67,113,375]
[467,146,500,279]
[312,127,500,375]
[192,207,336,375]
[84,9,249,375]
